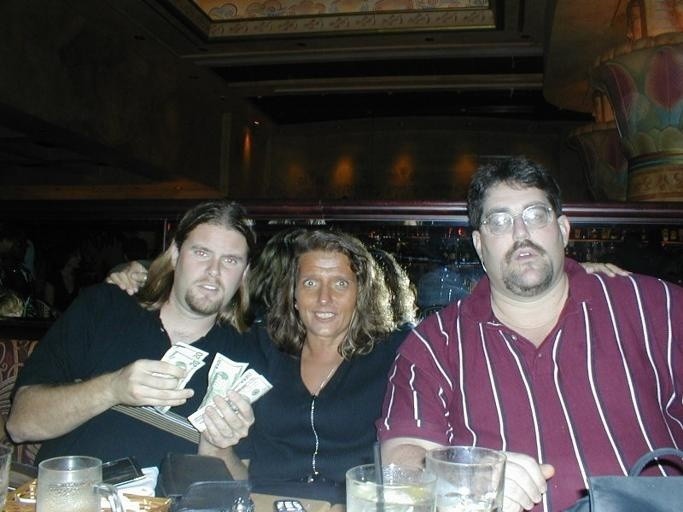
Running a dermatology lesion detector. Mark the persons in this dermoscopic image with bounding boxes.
[1,157,683,511]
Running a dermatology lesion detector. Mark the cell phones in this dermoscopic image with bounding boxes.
[274,499,306,512]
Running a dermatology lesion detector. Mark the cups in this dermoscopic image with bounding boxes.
[35,456,126,512]
[345,446,507,512]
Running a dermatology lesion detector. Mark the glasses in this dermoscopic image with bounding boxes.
[479,202,556,239]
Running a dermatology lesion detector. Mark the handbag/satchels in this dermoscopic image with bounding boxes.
[558,447,683,512]
